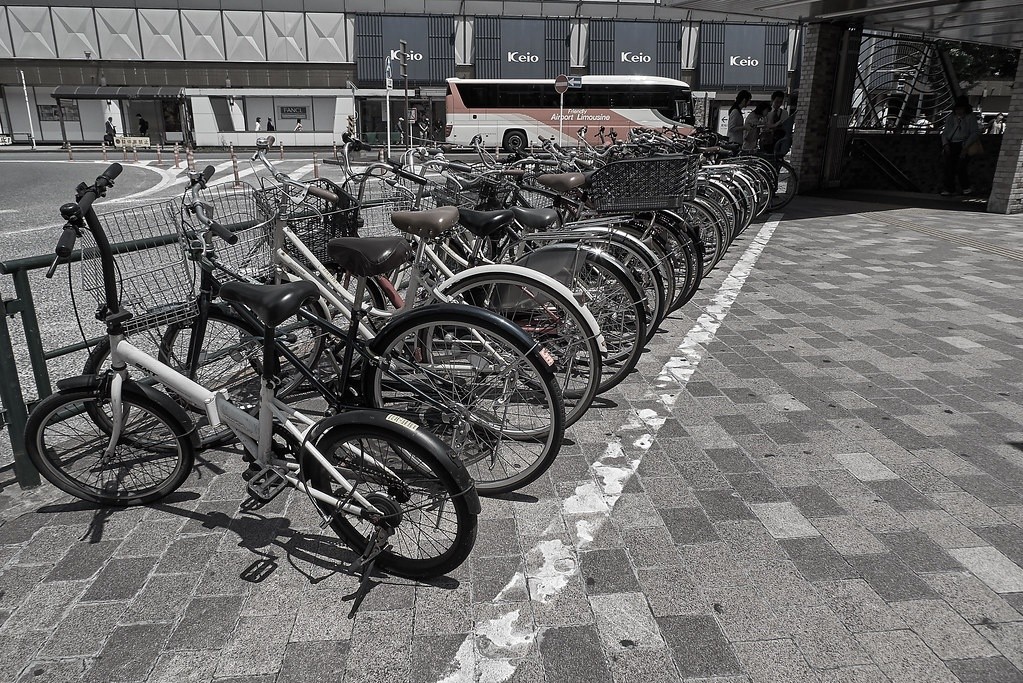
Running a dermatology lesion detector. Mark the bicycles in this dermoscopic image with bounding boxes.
[22,160,484,617]
[156,135,610,444]
[81,163,568,499]
[242,118,803,469]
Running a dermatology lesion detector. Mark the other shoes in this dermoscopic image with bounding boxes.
[941,191,958,195]
[963,184,976,195]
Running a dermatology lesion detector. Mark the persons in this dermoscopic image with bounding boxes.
[136,114,147,137]
[106,117,116,146]
[976,112,1007,134]
[764,90,789,132]
[727,90,752,147]
[293,118,302,132]
[418,119,428,146]
[255,117,262,131]
[941,96,978,196]
[267,117,274,131]
[742,101,771,150]
[396,117,405,144]
[909,111,934,134]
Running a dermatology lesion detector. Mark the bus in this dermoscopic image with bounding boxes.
[444,74,698,154]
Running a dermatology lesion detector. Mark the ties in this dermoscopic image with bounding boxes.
[774,109,779,123]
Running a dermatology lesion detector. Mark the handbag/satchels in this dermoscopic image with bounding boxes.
[967,138,983,155]
[759,128,786,154]
[742,128,757,149]
[936,149,945,166]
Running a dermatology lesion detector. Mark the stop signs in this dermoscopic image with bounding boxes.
[554,74,570,94]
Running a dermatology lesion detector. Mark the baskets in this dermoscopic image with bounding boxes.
[591,154,699,211]
[81,142,600,335]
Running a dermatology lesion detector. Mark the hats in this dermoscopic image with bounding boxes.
[952,95,973,109]
